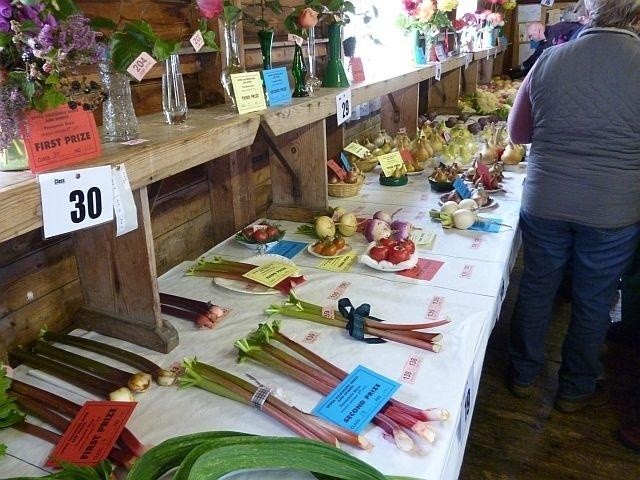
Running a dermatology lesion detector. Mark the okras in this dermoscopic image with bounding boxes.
[127,431,383,480]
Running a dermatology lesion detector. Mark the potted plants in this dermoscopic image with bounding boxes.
[255,2,283,107]
[317,0,356,89]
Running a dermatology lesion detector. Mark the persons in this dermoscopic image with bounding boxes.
[520,20,584,72]
[505,1,639,414]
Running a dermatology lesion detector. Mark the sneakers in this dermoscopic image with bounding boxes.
[619,425,640,452]
[554,380,617,412]
[510,380,534,398]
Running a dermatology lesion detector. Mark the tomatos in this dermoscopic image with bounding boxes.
[314,234,346,255]
[240,220,280,245]
[369,236,415,264]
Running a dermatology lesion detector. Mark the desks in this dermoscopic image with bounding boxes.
[0,96,533,480]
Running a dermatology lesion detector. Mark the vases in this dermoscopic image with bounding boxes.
[0,137,32,173]
[413,28,501,64]
[162,53,190,126]
[2,85,30,173]
[291,29,322,98]
[94,20,142,142]
[216,13,248,116]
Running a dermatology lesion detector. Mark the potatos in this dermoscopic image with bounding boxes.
[417,110,502,133]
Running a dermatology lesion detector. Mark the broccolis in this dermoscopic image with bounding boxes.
[467,86,498,115]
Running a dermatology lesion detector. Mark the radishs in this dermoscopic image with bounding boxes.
[296,205,369,238]
[429,198,514,230]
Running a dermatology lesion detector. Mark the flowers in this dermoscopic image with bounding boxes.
[0,1,108,110]
[298,8,319,29]
[400,1,523,31]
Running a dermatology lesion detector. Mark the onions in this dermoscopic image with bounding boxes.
[327,116,526,188]
[364,207,423,239]
